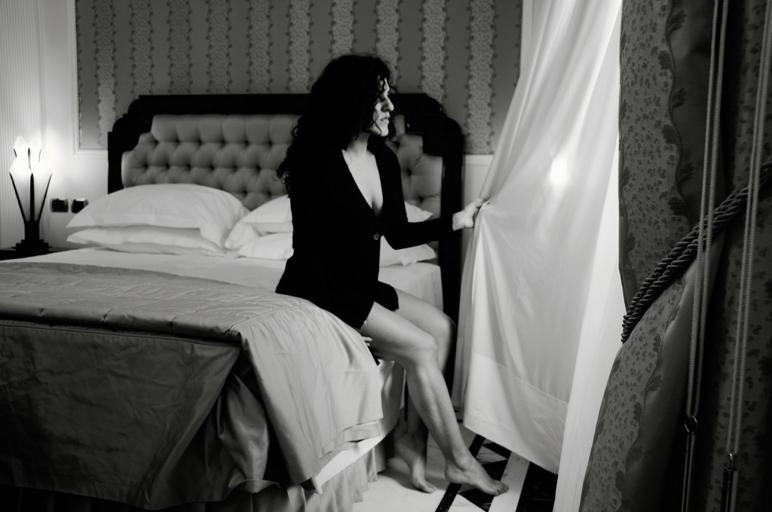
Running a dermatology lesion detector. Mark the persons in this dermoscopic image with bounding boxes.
[275,55,511,496]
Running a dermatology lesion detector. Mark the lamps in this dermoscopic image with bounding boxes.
[6,128,65,253]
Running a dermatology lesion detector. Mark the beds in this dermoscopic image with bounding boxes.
[0,92,467,512]
